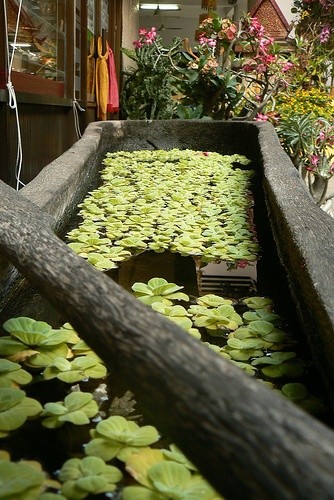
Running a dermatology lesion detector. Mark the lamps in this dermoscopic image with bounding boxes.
[153,0,162,16]
